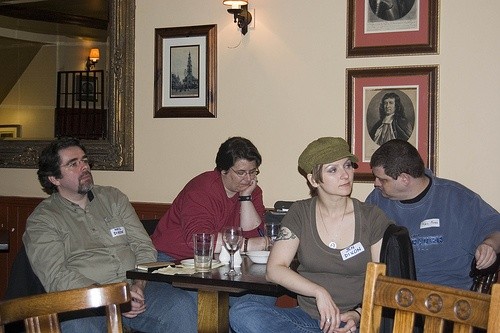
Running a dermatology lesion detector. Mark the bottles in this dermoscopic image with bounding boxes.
[219,246,230,265]
[233,250,242,267]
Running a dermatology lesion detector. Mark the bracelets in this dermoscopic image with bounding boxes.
[238,195,252,201]
[354,309,361,317]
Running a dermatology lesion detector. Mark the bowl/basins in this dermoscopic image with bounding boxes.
[246,249,271,263]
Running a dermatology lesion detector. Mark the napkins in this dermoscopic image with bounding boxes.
[152,265,198,275]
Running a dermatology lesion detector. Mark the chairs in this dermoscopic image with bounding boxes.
[0,283,131,333]
[9,245,46,297]
[359,226,500,333]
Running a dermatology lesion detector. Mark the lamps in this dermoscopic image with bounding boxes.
[86,48,100,68]
[223,0,252,36]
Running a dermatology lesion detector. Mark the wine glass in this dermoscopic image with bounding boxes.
[222,226,242,276]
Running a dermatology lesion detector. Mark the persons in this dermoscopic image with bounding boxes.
[365,139,500,290]
[151,137,265,304]
[22,138,198,333]
[230,137,390,333]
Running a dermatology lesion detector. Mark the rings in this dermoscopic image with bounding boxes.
[326,319,330,322]
[349,329,351,331]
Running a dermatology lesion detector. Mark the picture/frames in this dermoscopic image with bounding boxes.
[76,75,98,102]
[346,64,439,182]
[346,0,440,58]
[0,124,22,137]
[153,24,217,118]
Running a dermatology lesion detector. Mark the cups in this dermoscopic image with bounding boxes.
[193,233,215,273]
[264,222,281,252]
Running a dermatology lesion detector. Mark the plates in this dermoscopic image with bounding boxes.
[180,259,220,267]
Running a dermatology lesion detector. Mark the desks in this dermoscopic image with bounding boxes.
[129,253,287,333]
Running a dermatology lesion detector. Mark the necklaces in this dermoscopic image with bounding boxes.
[318,197,347,250]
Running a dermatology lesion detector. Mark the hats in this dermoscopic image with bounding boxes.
[298,137,359,174]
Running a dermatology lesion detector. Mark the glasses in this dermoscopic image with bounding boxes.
[58,160,88,170]
[230,166,260,178]
